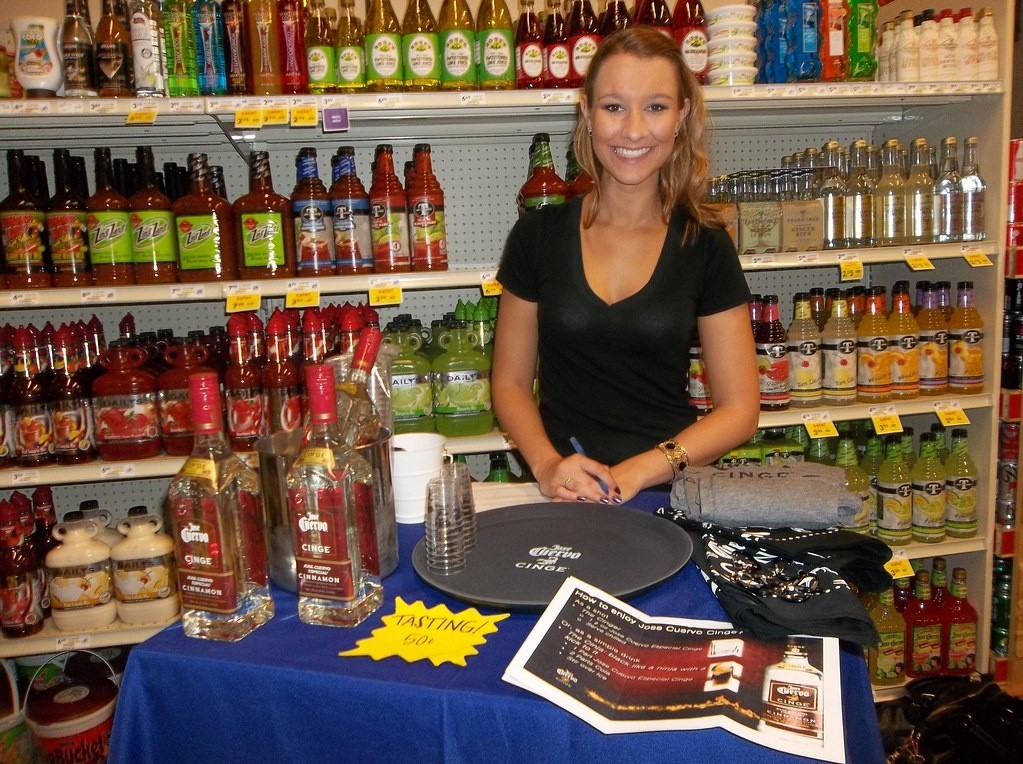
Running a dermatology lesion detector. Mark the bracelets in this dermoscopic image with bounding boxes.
[655,439,692,487]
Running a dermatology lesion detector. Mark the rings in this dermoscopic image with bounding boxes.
[564,478,573,487]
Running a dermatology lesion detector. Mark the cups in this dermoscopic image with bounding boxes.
[389,430,447,524]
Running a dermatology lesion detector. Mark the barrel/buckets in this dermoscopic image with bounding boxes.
[0,648,127,763]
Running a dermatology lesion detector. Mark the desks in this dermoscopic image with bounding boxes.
[105,492,887,763]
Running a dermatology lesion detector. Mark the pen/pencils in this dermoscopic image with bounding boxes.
[570,436,610,495]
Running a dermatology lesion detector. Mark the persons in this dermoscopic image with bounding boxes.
[494,27,761,506]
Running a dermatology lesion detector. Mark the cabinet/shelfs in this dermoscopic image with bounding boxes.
[0,0,1023,701]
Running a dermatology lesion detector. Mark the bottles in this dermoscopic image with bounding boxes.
[0,0,1023,741]
